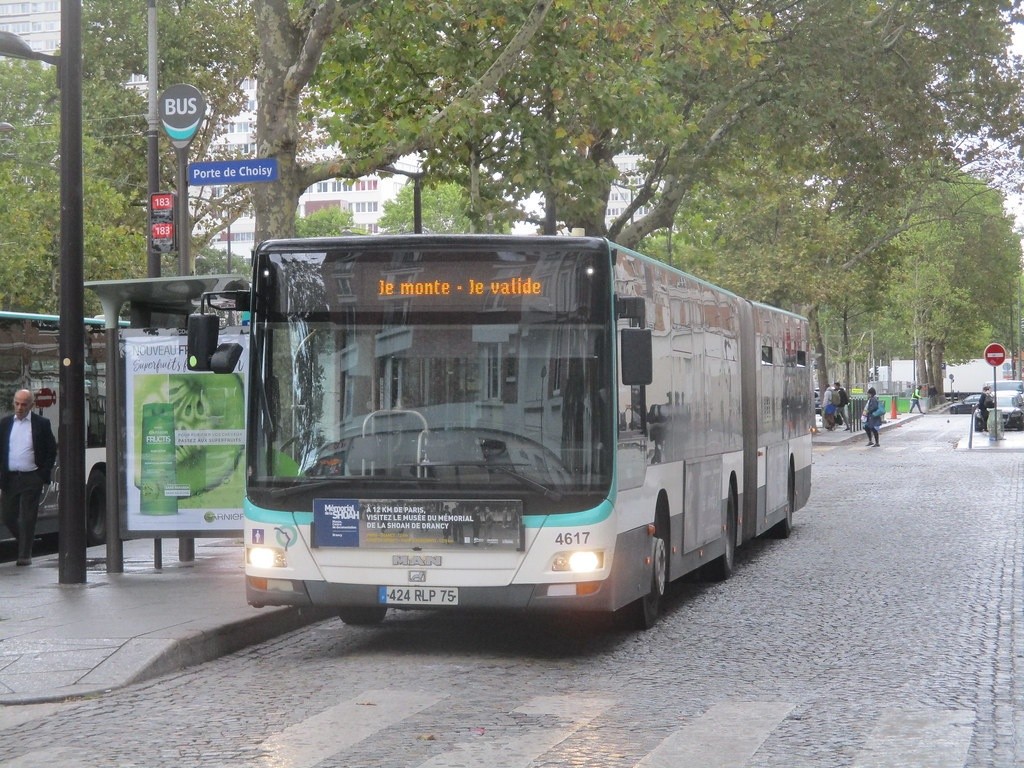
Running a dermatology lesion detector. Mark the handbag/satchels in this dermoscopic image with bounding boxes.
[984,394,995,408]
[868,397,886,417]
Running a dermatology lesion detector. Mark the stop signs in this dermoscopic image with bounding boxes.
[983,343,1006,367]
[35,387,54,407]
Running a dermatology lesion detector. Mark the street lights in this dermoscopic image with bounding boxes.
[1,2,97,588]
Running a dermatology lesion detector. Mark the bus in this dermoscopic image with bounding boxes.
[184,227,825,631]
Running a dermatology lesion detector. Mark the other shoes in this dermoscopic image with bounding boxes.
[866,441,874,446]
[16,557,32,565]
[872,444,880,447]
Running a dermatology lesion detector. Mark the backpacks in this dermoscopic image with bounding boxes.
[827,389,840,406]
[836,387,849,405]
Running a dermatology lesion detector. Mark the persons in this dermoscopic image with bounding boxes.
[909,385,925,414]
[0,389,57,565]
[863,387,880,447]
[821,384,836,431]
[978,386,995,432]
[834,382,850,430]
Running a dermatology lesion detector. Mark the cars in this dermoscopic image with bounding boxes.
[986,380,1023,402]
[949,394,984,413]
[972,390,1024,431]
[812,387,822,414]
